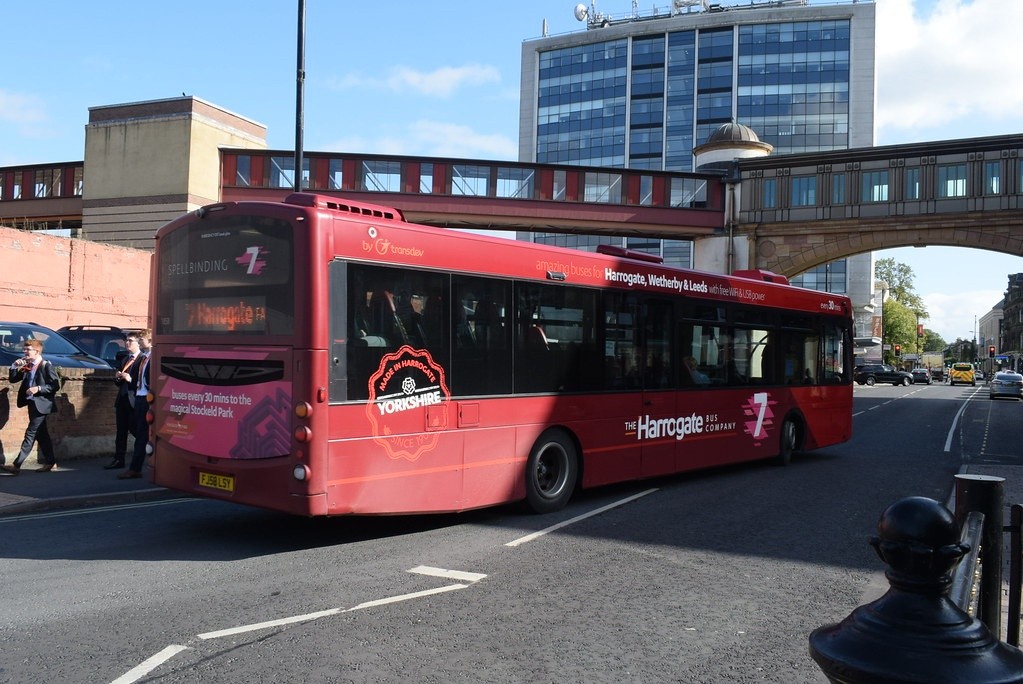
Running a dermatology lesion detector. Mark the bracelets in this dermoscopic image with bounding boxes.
[38,386,41,392]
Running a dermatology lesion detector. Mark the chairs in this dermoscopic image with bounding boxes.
[104,342,120,361]
[372,291,551,355]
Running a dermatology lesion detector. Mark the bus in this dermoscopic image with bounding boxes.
[146,192,852,517]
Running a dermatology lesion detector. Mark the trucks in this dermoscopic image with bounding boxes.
[921,351,943,381]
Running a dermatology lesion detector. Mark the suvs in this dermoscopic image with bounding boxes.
[950,363,975,386]
[54,324,150,376]
[854,364,915,387]
[973,370,984,379]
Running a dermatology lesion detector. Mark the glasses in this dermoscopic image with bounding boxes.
[125,339,137,343]
[23,348,35,352]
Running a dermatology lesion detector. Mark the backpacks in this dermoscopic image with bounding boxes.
[41,360,65,394]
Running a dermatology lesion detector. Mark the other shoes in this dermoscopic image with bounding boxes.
[35,463,58,473]
[103,459,125,470]
[116,470,141,480]
[1,465,20,474]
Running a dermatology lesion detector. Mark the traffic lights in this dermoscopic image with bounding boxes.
[894,344,901,356]
[989,345,996,358]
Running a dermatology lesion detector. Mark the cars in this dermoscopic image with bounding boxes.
[0,320,112,374]
[989,370,1023,400]
[911,368,933,384]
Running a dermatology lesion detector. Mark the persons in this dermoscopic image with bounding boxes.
[0,339,61,474]
[103,328,153,479]
